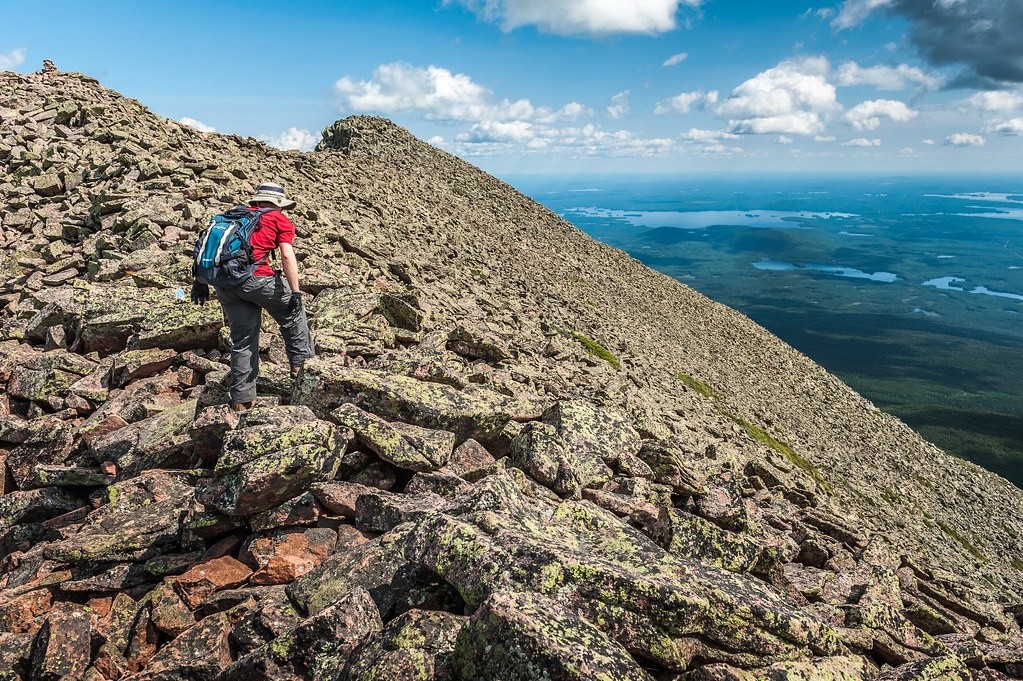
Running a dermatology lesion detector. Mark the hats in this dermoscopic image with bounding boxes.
[248,182,297,210]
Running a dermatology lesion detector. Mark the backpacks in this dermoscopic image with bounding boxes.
[192,204,277,289]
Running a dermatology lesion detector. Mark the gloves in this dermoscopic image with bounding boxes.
[190,279,209,306]
[282,292,304,330]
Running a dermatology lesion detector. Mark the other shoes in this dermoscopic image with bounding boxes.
[231,399,256,414]
[290,367,300,378]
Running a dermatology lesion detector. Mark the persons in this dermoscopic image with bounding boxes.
[188,181,314,412]
